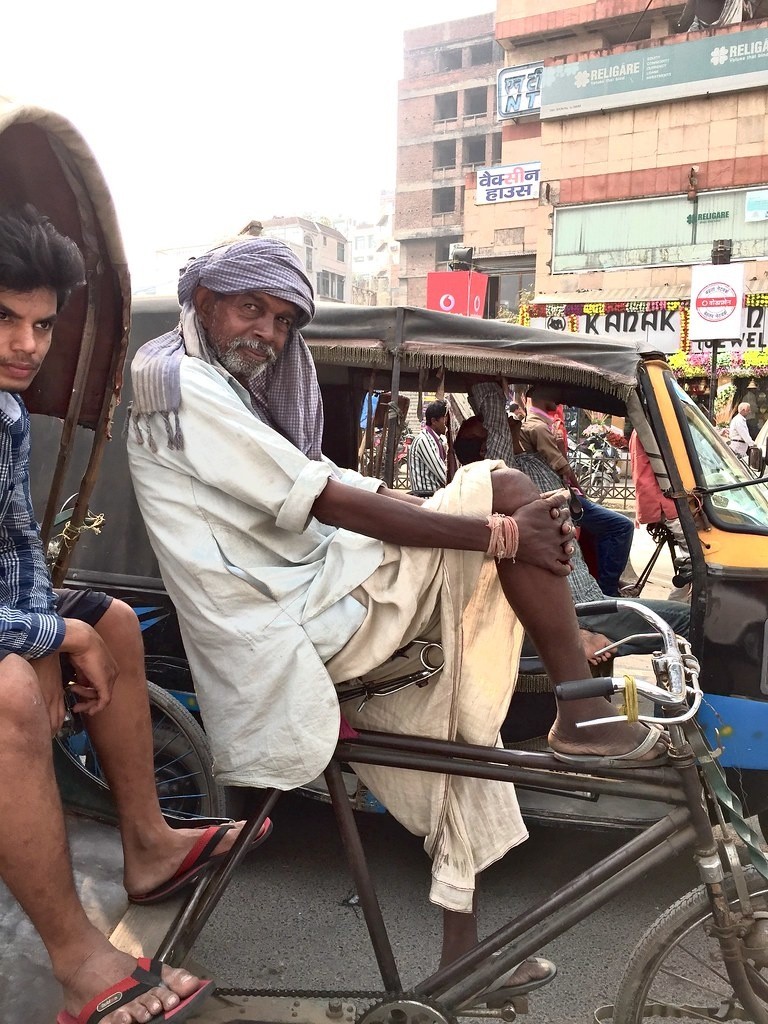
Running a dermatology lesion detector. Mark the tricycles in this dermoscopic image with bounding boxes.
[0,111,768,1023]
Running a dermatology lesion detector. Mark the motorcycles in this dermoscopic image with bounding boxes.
[31,297,766,852]
[567,431,622,502]
[359,421,415,473]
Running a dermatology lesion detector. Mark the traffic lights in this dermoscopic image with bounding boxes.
[685,163,702,207]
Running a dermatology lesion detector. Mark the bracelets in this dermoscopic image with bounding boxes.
[484,513,519,558]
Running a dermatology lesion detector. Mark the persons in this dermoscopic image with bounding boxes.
[521,388,690,599]
[409,398,526,502]
[121,234,675,1009]
[729,381,768,457]
[455,380,692,665]
[0,203,273,1024]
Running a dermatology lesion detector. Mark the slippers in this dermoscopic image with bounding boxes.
[553,719,673,768]
[454,955,557,1016]
[57,957,217,1024]
[127,815,274,905]
